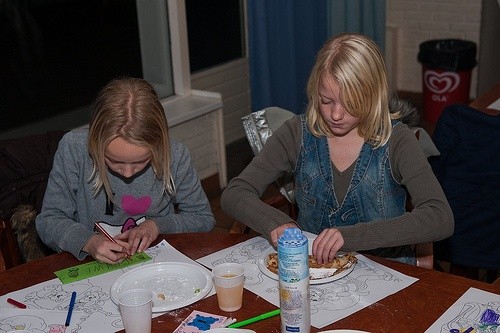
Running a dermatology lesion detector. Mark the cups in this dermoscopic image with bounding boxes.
[118,290,153,333]
[211,263,245,312]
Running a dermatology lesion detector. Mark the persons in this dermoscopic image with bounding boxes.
[220,34,454,265]
[36,80,216,265]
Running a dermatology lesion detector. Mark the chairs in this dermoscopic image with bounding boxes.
[428,105,500,283]
[0,131,71,270]
[229,107,441,270]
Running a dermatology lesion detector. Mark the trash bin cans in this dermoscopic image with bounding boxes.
[420,39,477,122]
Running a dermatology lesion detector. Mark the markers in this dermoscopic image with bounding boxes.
[94,222,133,262]
[226,309,280,328]
[65,291,76,326]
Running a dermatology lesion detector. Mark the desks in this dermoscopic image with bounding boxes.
[0,233,500,333]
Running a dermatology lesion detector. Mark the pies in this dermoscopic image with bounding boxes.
[266,252,357,281]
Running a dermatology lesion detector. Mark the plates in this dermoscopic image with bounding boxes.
[258,236,356,285]
[110,261,213,312]
